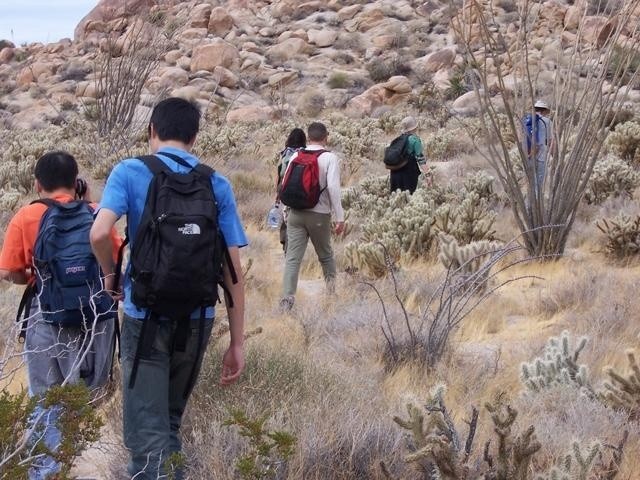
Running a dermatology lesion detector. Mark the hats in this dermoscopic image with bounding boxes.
[533,101,550,109]
[399,116,418,133]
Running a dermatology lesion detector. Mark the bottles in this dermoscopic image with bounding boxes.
[268,205,280,228]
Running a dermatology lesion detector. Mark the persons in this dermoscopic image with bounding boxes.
[90,97,248,479]
[272,122,344,314]
[277,129,310,258]
[389,116,428,198]
[0,150,124,480]
[523,100,552,198]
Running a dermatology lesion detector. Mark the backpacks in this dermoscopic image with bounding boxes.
[523,113,548,158]
[383,133,416,169]
[275,147,331,211]
[16,198,119,344]
[112,154,239,389]
